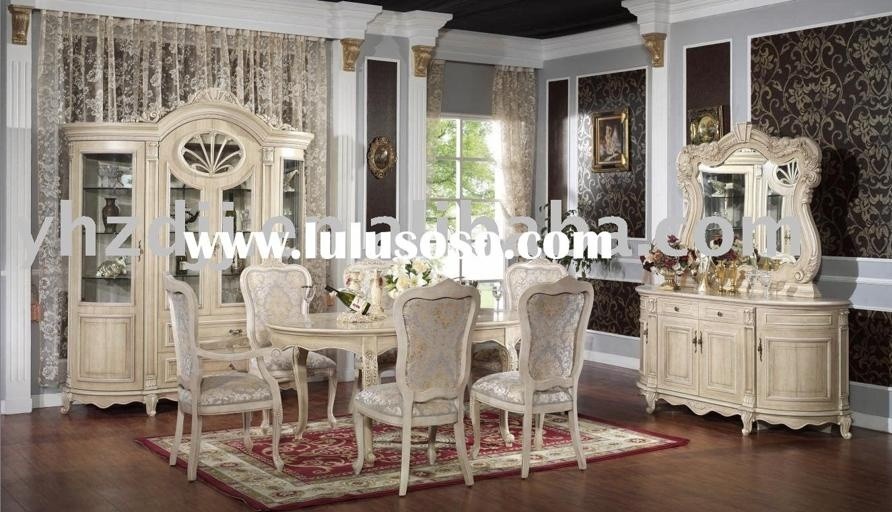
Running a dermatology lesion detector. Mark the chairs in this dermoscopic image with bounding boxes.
[164,274,284,482]
[349,276,479,498]
[238,258,341,440]
[467,274,596,481]
[466,256,572,374]
[343,259,399,416]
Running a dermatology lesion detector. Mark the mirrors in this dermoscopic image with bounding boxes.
[676,121,824,299]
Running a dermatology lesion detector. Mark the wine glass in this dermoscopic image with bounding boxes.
[744,269,772,300]
[491,285,503,314]
[96,160,120,188]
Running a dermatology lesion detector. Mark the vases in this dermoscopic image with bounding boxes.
[658,269,680,292]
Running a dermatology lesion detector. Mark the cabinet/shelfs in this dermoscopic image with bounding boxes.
[634,288,658,393]
[61,87,314,418]
[657,292,754,412]
[695,149,791,255]
[754,303,851,417]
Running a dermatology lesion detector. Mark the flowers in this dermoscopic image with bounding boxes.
[382,255,443,301]
[639,234,701,278]
[710,234,740,265]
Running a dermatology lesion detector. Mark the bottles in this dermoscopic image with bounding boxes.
[717,260,737,292]
[230,250,245,274]
[226,192,237,232]
[321,284,371,315]
[176,247,188,275]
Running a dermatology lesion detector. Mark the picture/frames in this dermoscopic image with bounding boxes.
[591,105,632,172]
[687,105,724,146]
[366,135,397,180]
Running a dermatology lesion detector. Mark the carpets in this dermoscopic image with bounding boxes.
[132,396,691,512]
